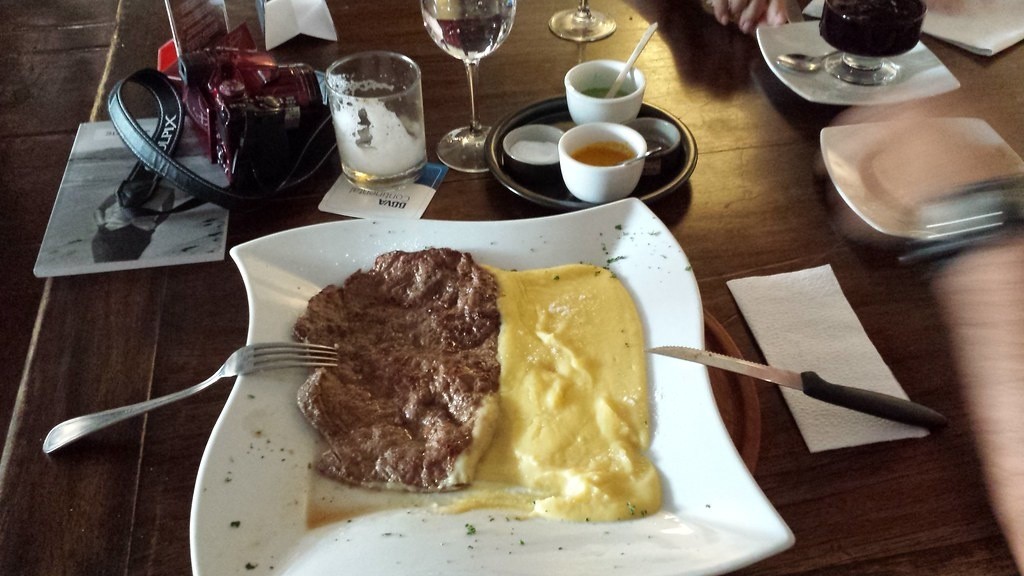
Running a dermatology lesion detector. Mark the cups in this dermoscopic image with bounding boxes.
[557,121,647,204]
[819,0,928,85]
[564,58,647,125]
[324,50,428,192]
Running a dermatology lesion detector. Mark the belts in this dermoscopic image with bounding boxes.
[108,65,337,220]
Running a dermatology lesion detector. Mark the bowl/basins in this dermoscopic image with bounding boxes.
[619,117,682,160]
[502,123,567,173]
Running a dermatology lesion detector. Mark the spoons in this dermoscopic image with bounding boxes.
[774,52,844,72]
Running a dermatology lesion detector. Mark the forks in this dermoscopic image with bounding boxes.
[41,341,338,454]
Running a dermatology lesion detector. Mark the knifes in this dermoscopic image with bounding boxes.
[642,345,949,432]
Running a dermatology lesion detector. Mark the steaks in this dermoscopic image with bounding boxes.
[292,248,501,491]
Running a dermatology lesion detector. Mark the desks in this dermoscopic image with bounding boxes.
[0,0,1024,576]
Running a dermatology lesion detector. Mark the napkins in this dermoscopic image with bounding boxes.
[726,263,930,455]
[263,0,337,52]
[802,0,1024,57]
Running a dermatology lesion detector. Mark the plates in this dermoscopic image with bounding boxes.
[820,117,1024,240]
[756,20,961,106]
[189,196,797,576]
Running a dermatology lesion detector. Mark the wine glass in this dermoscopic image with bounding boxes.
[420,0,517,173]
[547,0,617,42]
